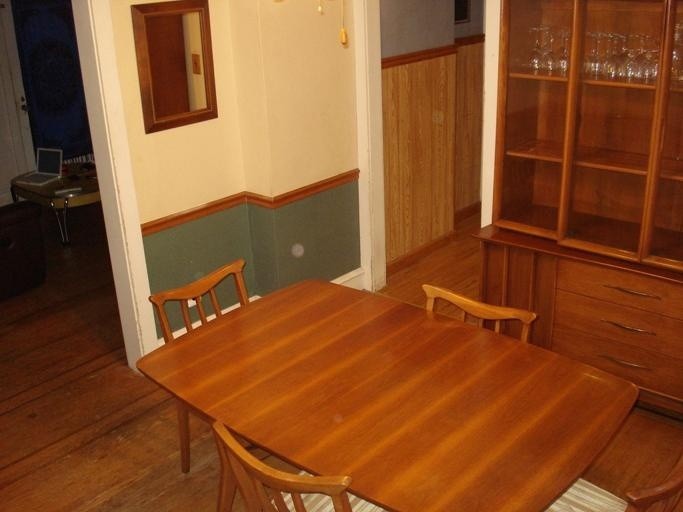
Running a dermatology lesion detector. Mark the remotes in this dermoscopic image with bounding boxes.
[54,185,82,195]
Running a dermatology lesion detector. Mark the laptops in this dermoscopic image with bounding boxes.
[15,148,63,187]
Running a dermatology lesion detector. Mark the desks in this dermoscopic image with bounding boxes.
[135,278,640,510]
[9,169,100,244]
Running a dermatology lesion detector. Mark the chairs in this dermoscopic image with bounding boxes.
[0,199,48,304]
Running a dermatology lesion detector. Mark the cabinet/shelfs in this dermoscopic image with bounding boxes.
[469,2,683,426]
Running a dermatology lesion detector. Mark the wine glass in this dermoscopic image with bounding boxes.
[527,23,682,82]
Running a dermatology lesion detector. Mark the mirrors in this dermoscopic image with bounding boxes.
[131,0,218,136]
